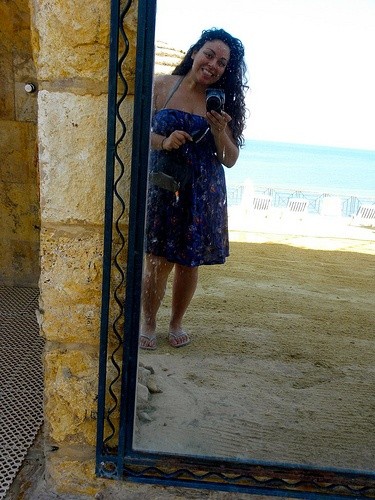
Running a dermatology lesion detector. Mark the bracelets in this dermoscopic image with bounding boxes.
[161,137,167,151]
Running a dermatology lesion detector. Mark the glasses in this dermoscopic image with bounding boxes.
[177,127,211,157]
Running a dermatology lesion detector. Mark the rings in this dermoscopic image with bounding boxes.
[218,128,221,132]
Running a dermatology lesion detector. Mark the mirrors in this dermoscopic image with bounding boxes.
[99,0,375,500]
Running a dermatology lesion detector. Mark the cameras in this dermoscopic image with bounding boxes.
[205,88,225,117]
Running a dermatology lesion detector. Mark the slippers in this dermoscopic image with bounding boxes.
[139,333,157,350]
[168,330,191,348]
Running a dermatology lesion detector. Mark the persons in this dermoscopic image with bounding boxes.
[139,28,249,349]
